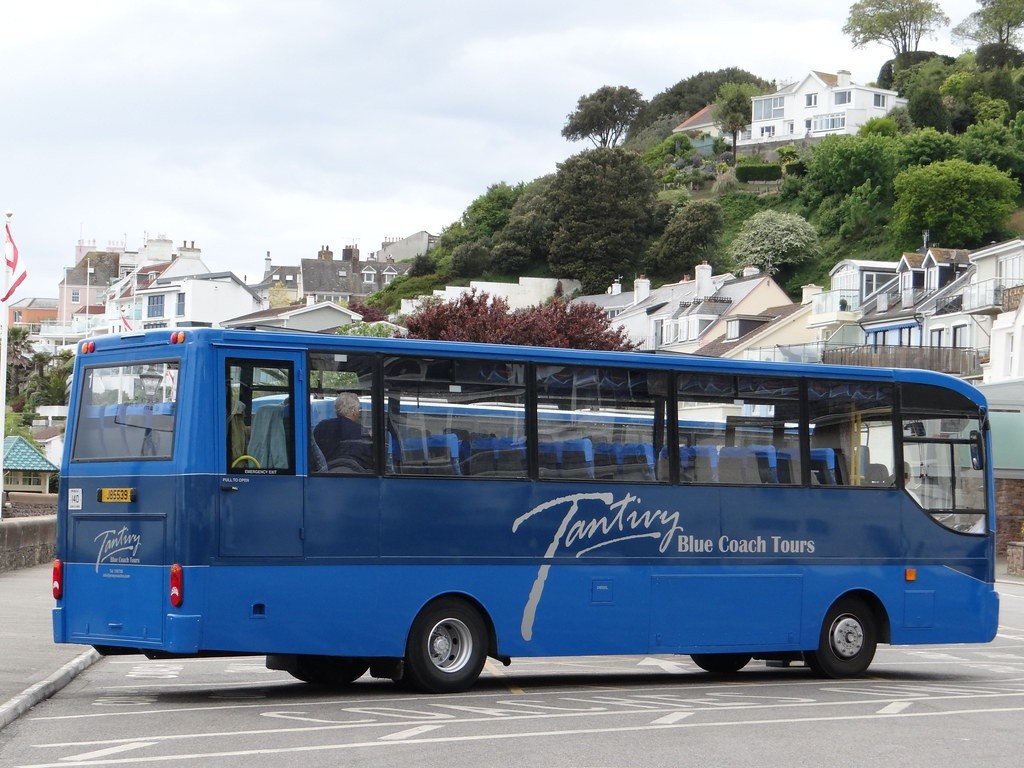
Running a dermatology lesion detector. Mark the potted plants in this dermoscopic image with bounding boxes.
[839,299,847,311]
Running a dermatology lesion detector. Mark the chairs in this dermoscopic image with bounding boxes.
[400,436,431,474]
[692,445,718,483]
[776,449,801,484]
[562,438,595,479]
[428,433,463,477]
[870,464,889,483]
[594,444,622,480]
[469,437,497,477]
[748,444,778,483]
[538,441,562,477]
[718,447,761,483]
[811,448,836,485]
[622,443,656,481]
[658,447,697,481]
[498,438,527,477]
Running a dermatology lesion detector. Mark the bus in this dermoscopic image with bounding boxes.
[251,394,872,468]
[52,327,1000,696]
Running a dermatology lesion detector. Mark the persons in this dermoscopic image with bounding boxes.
[891,461,923,508]
[313,392,373,469]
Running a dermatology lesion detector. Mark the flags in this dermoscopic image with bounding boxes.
[0,222,28,303]
